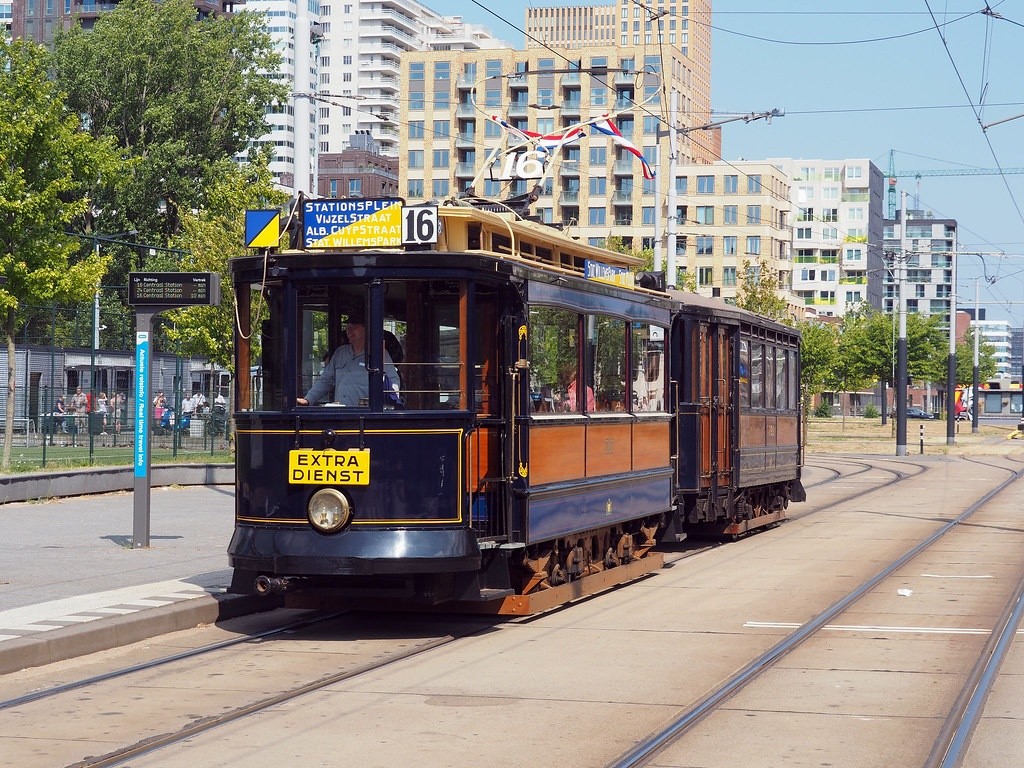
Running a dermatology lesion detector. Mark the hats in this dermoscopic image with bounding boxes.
[341,312,364,324]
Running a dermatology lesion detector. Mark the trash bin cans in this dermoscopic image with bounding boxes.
[89,410,104,436]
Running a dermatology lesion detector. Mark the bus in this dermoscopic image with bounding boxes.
[226,199,807,619]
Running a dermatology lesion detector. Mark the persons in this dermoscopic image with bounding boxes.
[201,402,210,413]
[214,392,225,407]
[70,387,87,433]
[54,393,68,434]
[97,392,108,435]
[153,391,167,426]
[538,360,594,413]
[182,393,195,418]
[193,389,205,413]
[85,390,96,414]
[110,392,124,436]
[297,311,400,406]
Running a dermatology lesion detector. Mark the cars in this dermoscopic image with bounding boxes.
[907,408,935,419]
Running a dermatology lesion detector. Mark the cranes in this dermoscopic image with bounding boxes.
[878,149,1024,221]
